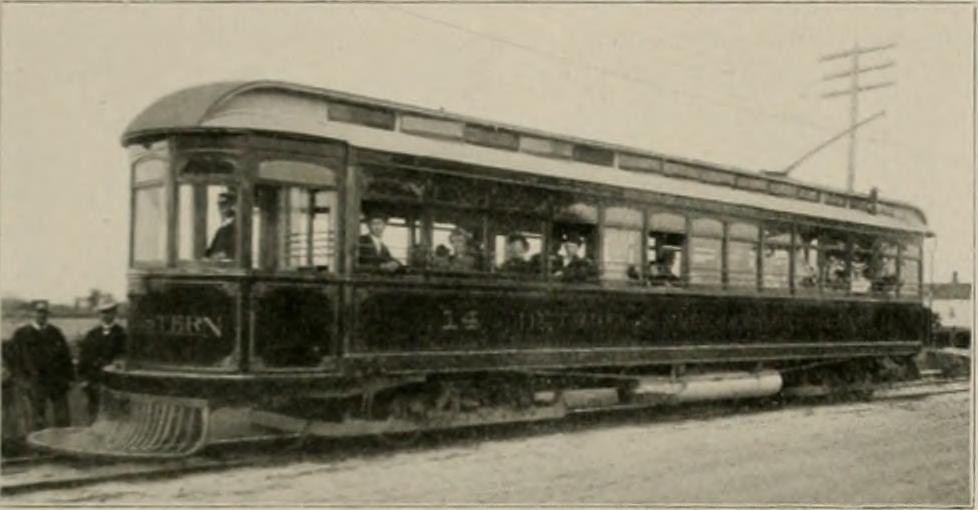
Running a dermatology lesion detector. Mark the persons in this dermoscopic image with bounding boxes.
[432,227,596,284]
[201,192,248,264]
[358,210,401,273]
[649,250,679,278]
[4,298,76,434]
[78,301,128,427]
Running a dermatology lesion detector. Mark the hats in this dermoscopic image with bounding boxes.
[96,298,118,310]
[217,193,236,204]
[561,233,582,247]
[361,207,389,222]
[34,302,47,311]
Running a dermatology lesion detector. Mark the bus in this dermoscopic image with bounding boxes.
[24,80,937,459]
